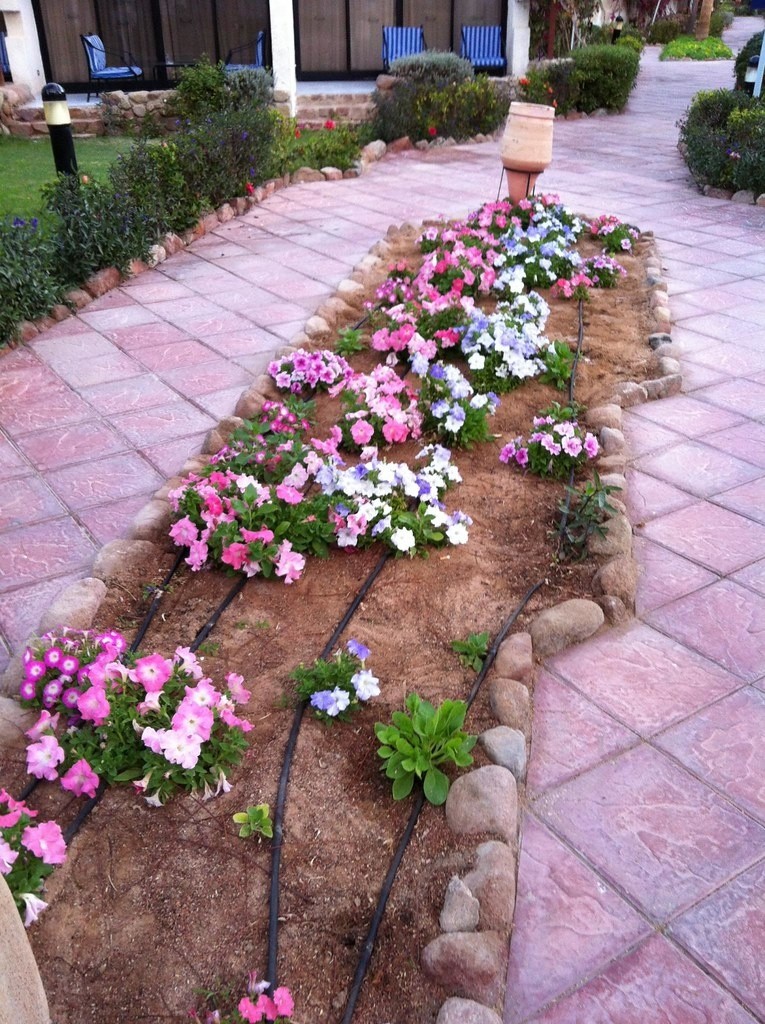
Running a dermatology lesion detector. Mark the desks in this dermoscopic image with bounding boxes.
[152,62,197,87]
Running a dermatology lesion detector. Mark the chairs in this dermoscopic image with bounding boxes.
[79,31,142,100]
[221,30,265,71]
[380,24,425,68]
[460,24,505,72]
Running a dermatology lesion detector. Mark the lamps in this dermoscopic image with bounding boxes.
[42,82,71,127]
[613,17,623,30]
[744,55,759,82]
